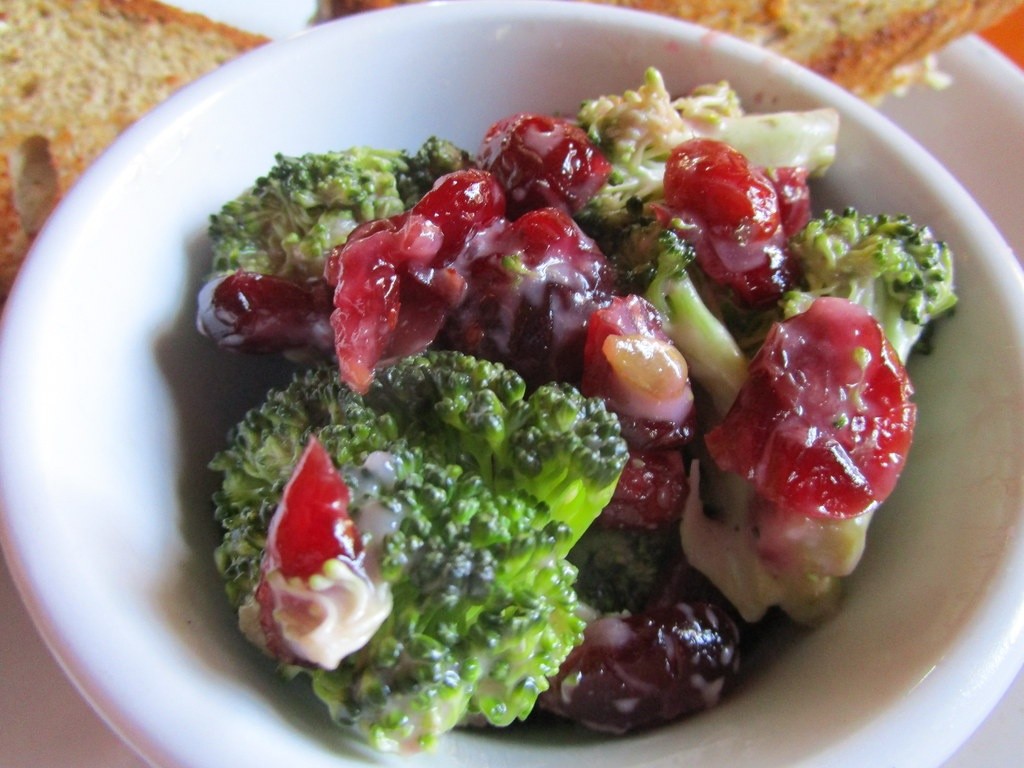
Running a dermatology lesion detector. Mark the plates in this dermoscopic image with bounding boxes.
[6,0,1019,768]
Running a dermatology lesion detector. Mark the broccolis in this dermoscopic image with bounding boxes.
[153,63,960,756]
